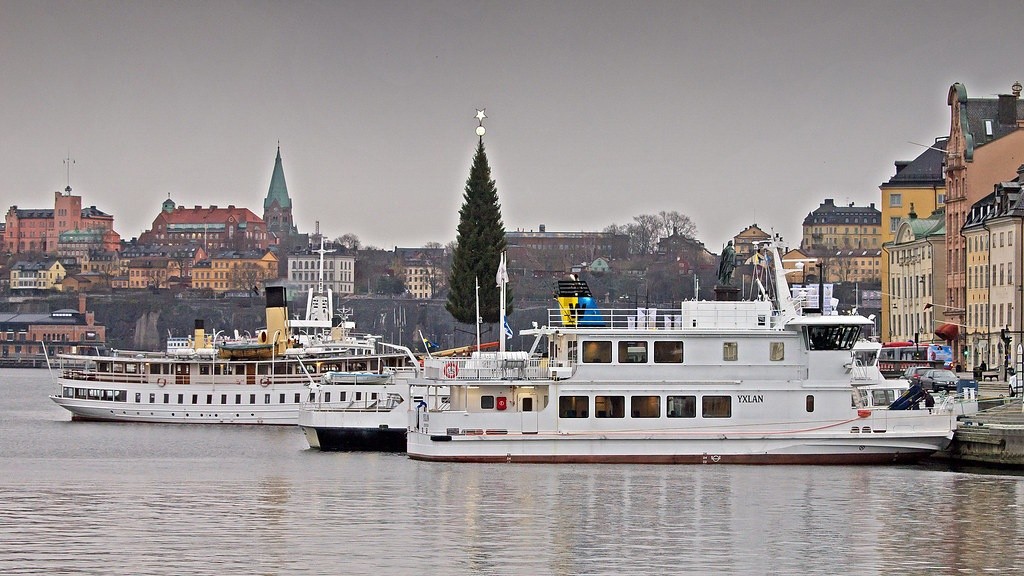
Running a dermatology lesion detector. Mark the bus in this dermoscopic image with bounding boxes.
[876,346,954,371]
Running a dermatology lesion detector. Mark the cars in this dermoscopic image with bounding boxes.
[920,370,960,393]
[1009,374,1023,397]
[904,367,934,385]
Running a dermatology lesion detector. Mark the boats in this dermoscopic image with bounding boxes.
[297,276,577,451]
[49,238,392,426]
[407,279,957,464]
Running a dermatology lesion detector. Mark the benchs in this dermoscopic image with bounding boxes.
[984,375,999,381]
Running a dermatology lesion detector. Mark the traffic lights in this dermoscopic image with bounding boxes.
[915,333,918,343]
[964,351,968,355]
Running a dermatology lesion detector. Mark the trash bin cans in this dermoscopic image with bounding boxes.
[955,380,979,395]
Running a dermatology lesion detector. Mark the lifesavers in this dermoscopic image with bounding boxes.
[444,362,459,379]
[259,377,270,387]
[157,377,166,387]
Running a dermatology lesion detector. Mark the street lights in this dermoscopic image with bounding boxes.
[1004,328,1012,382]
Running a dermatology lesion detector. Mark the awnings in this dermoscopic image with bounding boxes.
[935,324,958,341]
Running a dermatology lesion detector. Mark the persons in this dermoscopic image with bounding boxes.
[716,240,737,287]
[980,361,987,371]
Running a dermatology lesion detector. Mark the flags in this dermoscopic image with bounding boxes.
[505,317,514,339]
[496,260,510,288]
[424,338,439,349]
[745,253,772,268]
